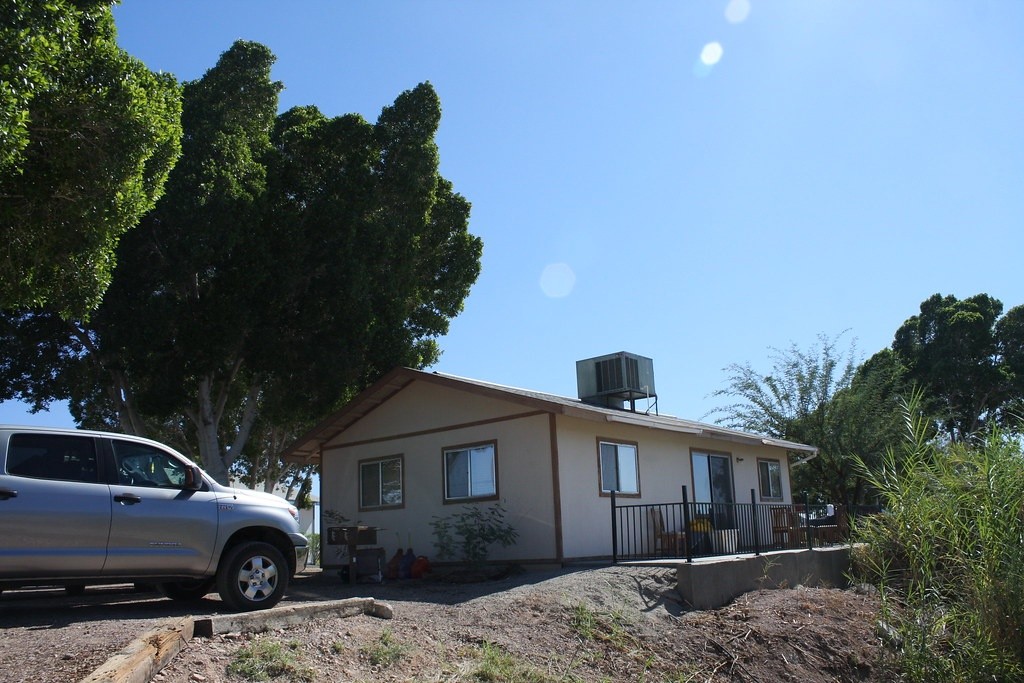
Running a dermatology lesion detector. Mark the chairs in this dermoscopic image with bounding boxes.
[45,444,80,480]
[647,507,686,557]
[770,507,804,549]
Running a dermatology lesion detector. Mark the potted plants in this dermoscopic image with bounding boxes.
[429,497,521,575]
[684,513,711,554]
[711,513,740,553]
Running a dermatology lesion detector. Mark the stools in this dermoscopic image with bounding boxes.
[799,525,837,548]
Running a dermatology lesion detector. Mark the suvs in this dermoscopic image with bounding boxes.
[0,424,309,615]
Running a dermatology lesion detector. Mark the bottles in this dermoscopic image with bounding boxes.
[399,548,417,579]
[411,556,431,578]
[387,549,404,580]
